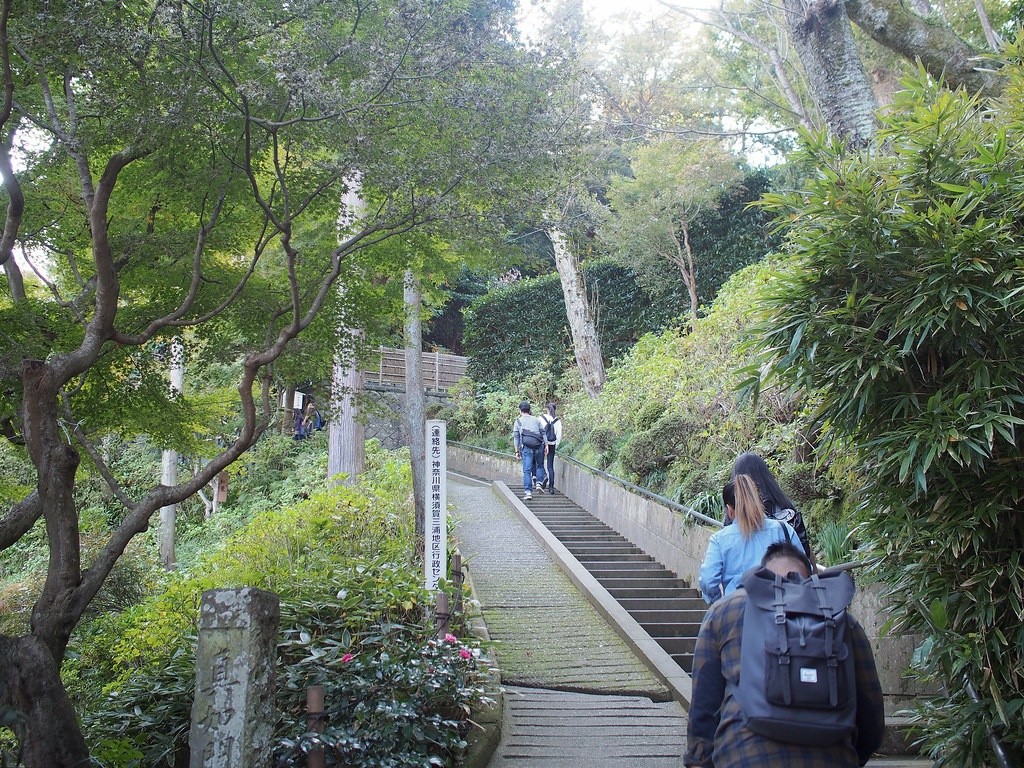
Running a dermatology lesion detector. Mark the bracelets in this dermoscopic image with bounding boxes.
[515,451,519,454]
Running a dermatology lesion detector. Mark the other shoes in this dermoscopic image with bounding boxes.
[549,489,554,494]
[523,493,532,500]
[536,484,545,494]
[532,476,537,486]
[542,474,548,489]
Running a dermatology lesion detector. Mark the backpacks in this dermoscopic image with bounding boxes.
[762,499,810,557]
[726,564,859,748]
[540,414,559,441]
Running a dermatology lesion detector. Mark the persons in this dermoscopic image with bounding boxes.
[686,542,884,768]
[292,395,321,440]
[513,402,563,500]
[724,453,818,574]
[699,476,812,604]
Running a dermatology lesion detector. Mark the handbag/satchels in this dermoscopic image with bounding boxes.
[521,429,543,449]
